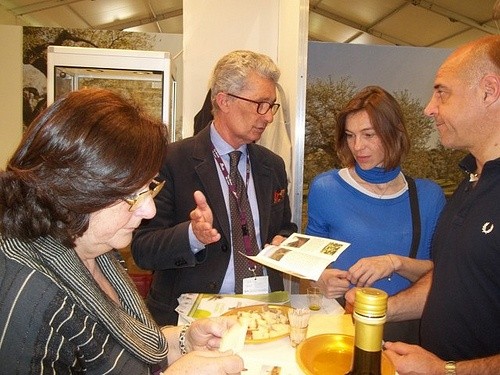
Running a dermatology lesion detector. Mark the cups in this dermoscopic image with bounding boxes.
[307,287,324,311]
[288,307,310,347]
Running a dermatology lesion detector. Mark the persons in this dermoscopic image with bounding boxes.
[298,86,445,346]
[130,53,298,327]
[344,36,500,375]
[0,88,245,375]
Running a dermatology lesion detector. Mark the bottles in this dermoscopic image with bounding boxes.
[343,287,389,375]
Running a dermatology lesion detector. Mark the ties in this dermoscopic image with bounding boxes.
[228,151,264,294]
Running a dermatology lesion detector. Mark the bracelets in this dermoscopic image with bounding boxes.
[179,324,189,357]
[445,361,456,375]
[386,254,395,281]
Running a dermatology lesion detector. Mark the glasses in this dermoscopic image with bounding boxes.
[121,179,165,212]
[225,94,280,115]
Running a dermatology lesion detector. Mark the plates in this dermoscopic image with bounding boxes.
[296,333,395,375]
[220,303,299,343]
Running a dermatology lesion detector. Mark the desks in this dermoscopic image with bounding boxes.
[177,294,399,375]
